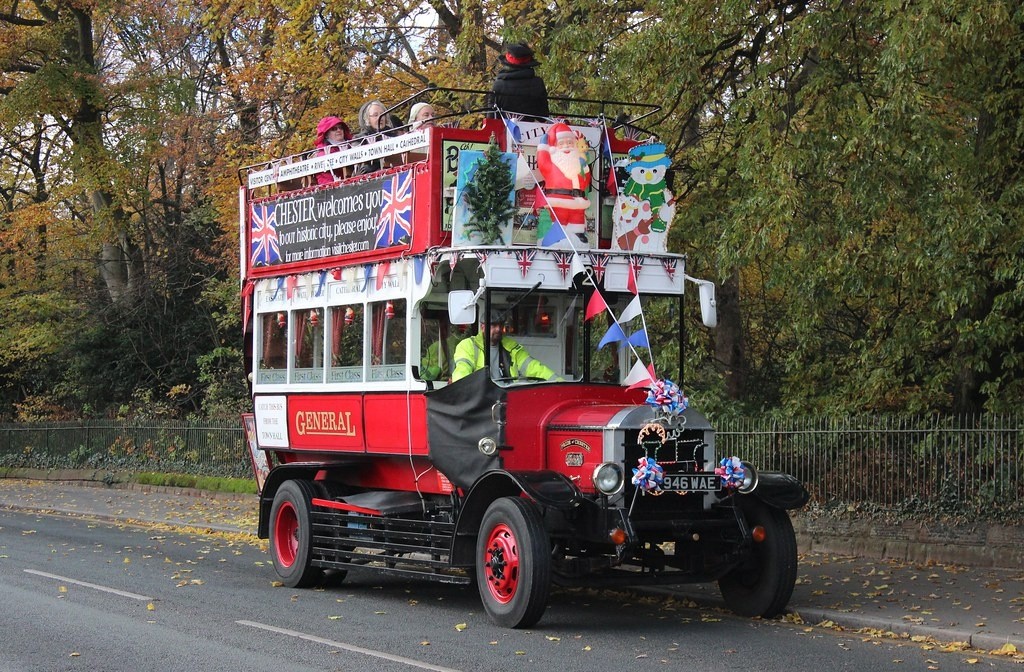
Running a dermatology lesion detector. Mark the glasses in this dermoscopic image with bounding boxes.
[328,126,344,131]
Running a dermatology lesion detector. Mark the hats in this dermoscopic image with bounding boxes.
[498,42,542,68]
[359,98,377,126]
[408,102,432,123]
[480,308,506,324]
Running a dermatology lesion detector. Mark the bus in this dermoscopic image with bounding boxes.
[238,84,816,631]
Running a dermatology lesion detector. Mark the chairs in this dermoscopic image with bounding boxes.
[269,146,427,192]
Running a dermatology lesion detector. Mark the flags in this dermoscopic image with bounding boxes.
[251,205,279,266]
[624,126,642,139]
[373,170,412,249]
[505,112,525,121]
[544,117,566,124]
[580,118,603,127]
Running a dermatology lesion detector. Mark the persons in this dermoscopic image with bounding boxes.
[351,100,398,177]
[420,324,470,381]
[488,41,549,122]
[390,115,404,137]
[537,123,590,242]
[451,310,565,386]
[313,117,353,184]
[408,103,435,130]
